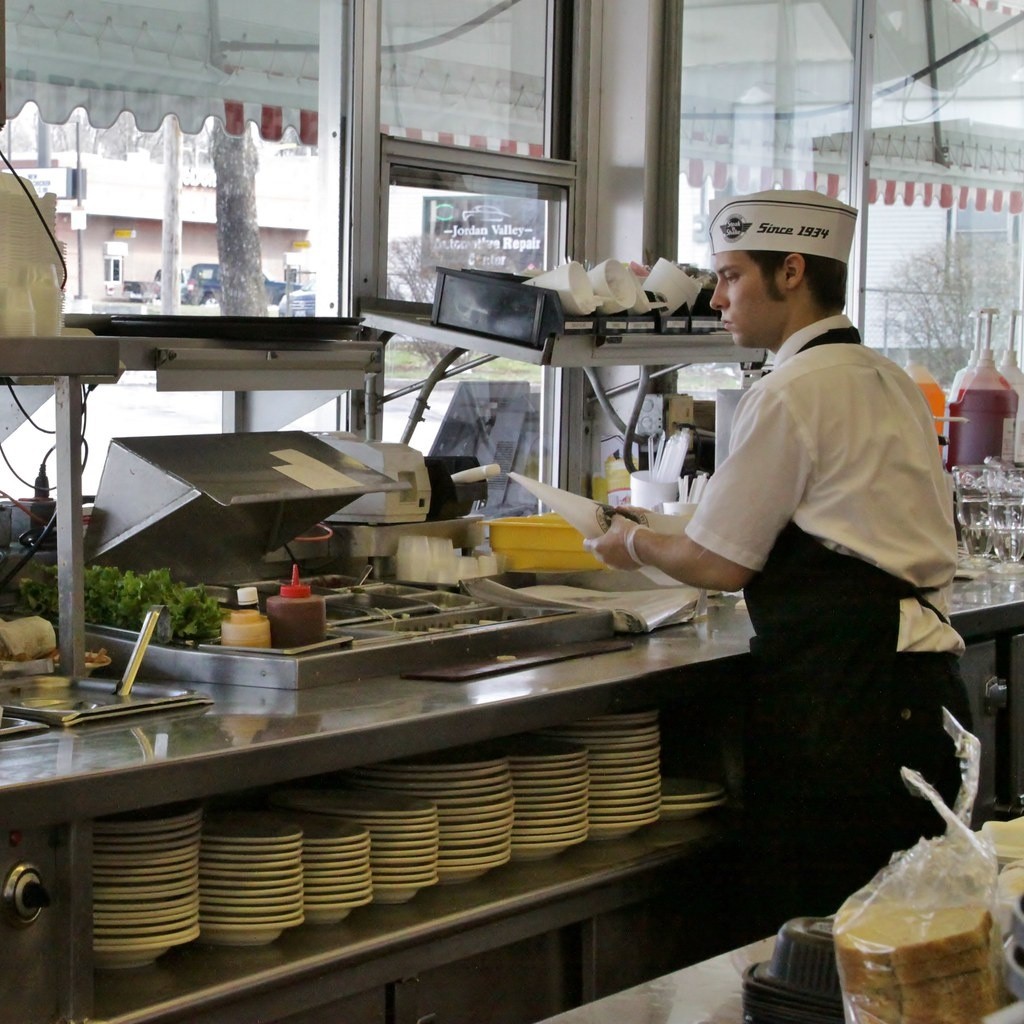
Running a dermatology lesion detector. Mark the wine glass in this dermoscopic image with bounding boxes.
[951,465,1024,575]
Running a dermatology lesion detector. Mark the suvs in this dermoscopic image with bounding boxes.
[183,261,304,305]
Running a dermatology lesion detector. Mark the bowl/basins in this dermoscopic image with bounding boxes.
[739,916,846,1024]
[479,515,609,571]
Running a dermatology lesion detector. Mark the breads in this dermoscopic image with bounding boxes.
[833,905,1012,1024]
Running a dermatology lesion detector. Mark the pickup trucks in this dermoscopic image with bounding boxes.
[122,269,191,303]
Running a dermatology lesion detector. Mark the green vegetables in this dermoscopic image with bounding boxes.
[10,561,231,644]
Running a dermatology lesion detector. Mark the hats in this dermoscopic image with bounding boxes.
[709,190,858,266]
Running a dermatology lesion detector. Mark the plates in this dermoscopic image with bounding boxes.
[90,702,726,970]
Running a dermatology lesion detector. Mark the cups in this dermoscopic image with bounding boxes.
[628,266,652,318]
[521,261,596,316]
[629,470,680,514]
[662,501,698,514]
[641,257,701,316]
[588,258,636,316]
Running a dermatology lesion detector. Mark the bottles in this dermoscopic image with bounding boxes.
[220,608,272,648]
[237,587,260,614]
[904,358,945,459]
[265,564,327,648]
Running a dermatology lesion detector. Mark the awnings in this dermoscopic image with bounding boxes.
[680,119,1024,213]
[3,0,547,158]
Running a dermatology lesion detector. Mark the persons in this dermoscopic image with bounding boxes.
[584,189,980,940]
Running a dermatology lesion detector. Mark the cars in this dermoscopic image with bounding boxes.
[279,278,317,318]
[462,205,512,225]
